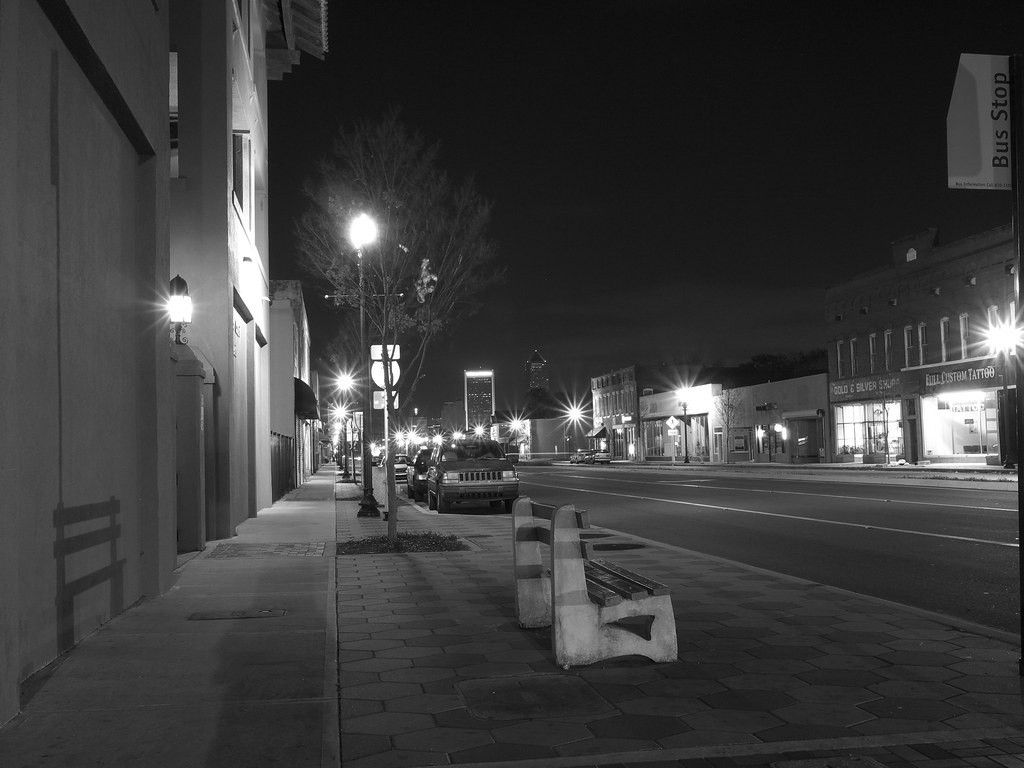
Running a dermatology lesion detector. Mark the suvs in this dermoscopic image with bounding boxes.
[425,438,520,514]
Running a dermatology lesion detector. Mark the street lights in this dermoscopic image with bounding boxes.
[332,374,351,480]
[678,387,692,463]
[988,324,1016,468]
[568,408,581,450]
[512,420,521,453]
[349,212,378,519]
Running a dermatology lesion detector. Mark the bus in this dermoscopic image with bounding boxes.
[369,439,391,466]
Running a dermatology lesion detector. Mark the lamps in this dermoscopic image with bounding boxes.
[169,272,193,344]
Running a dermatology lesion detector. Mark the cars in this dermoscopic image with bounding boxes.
[379,454,414,485]
[405,448,469,502]
[569,449,611,465]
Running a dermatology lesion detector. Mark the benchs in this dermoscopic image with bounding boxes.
[512,496,678,670]
